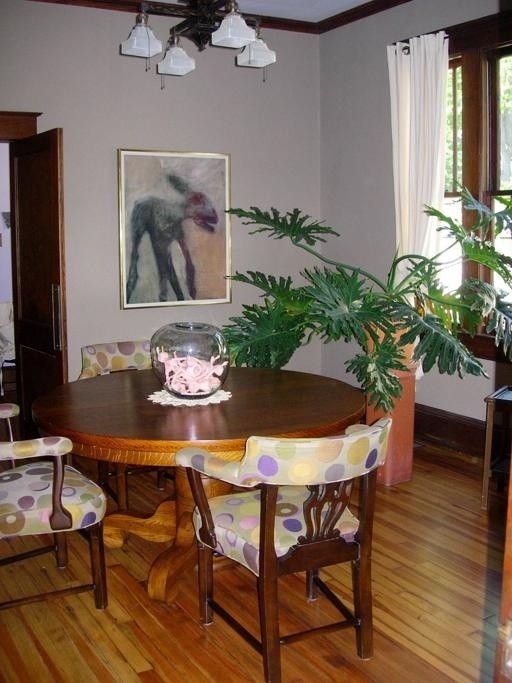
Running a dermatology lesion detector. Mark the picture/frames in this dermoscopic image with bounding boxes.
[117,148,232,309]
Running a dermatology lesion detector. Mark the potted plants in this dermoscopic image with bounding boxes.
[221,183,512,487]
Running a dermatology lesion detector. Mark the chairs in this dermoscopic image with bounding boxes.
[0,401,109,611]
[176,417,393,683]
[76,339,175,511]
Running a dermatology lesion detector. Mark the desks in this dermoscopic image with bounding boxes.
[480,384,512,511]
[30,365,368,604]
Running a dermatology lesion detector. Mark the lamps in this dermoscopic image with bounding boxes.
[121,0,277,90]
